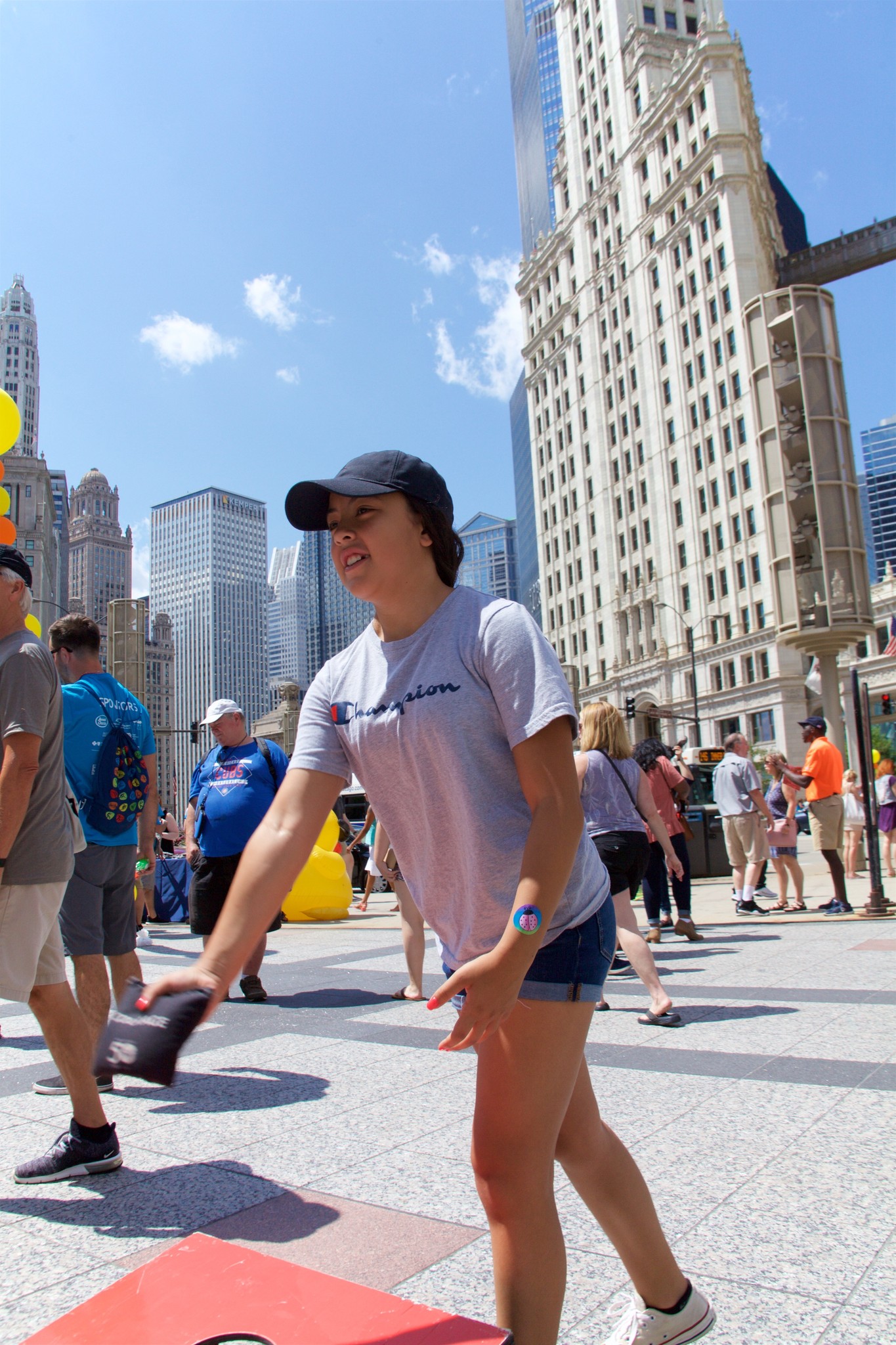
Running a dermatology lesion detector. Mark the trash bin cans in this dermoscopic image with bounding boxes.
[703,804,733,877]
[682,805,708,878]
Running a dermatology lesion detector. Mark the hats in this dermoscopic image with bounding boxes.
[199,699,242,727]
[285,450,454,531]
[796,716,827,732]
[0,543,32,588]
[338,819,350,842]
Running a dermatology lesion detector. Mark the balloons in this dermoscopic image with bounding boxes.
[0,387,42,638]
[872,749,881,775]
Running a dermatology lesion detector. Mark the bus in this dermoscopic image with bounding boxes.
[669,745,726,822]
[332,772,391,895]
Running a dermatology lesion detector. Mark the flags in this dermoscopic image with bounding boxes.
[804,655,823,696]
[882,615,896,656]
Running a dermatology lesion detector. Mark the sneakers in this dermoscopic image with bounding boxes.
[731,887,854,917]
[240,975,267,1002]
[14,1121,123,1184]
[138,924,143,931]
[597,1283,717,1344]
[145,915,170,925]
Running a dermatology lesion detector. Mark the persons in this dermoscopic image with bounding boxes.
[873,759,896,877]
[372,814,426,1000]
[579,738,704,975]
[331,794,363,902]
[347,791,401,911]
[715,716,868,918]
[185,698,283,1001]
[134,794,179,930]
[130,451,711,1345]
[566,701,682,1027]
[0,543,123,1188]
[32,611,158,1095]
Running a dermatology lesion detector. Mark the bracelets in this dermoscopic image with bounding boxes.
[353,831,357,835]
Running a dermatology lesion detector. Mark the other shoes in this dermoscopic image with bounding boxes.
[887,870,896,877]
[644,918,704,944]
[608,955,632,975]
[32,1073,114,1095]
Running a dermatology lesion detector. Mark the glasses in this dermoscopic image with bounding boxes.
[763,760,773,765]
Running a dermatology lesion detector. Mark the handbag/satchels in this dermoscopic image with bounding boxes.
[765,817,798,848]
[384,848,396,870]
[677,813,694,842]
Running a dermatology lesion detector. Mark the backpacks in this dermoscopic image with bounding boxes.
[64,683,150,838]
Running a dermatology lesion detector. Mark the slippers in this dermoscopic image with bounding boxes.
[595,1002,682,1025]
[391,986,427,1001]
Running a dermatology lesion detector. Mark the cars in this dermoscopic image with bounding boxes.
[795,813,811,835]
[174,845,187,855]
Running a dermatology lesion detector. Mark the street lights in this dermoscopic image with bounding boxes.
[654,601,725,878]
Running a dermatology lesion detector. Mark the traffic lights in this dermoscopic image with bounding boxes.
[881,693,892,715]
[625,697,635,719]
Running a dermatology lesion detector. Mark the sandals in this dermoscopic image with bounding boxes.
[352,902,401,912]
